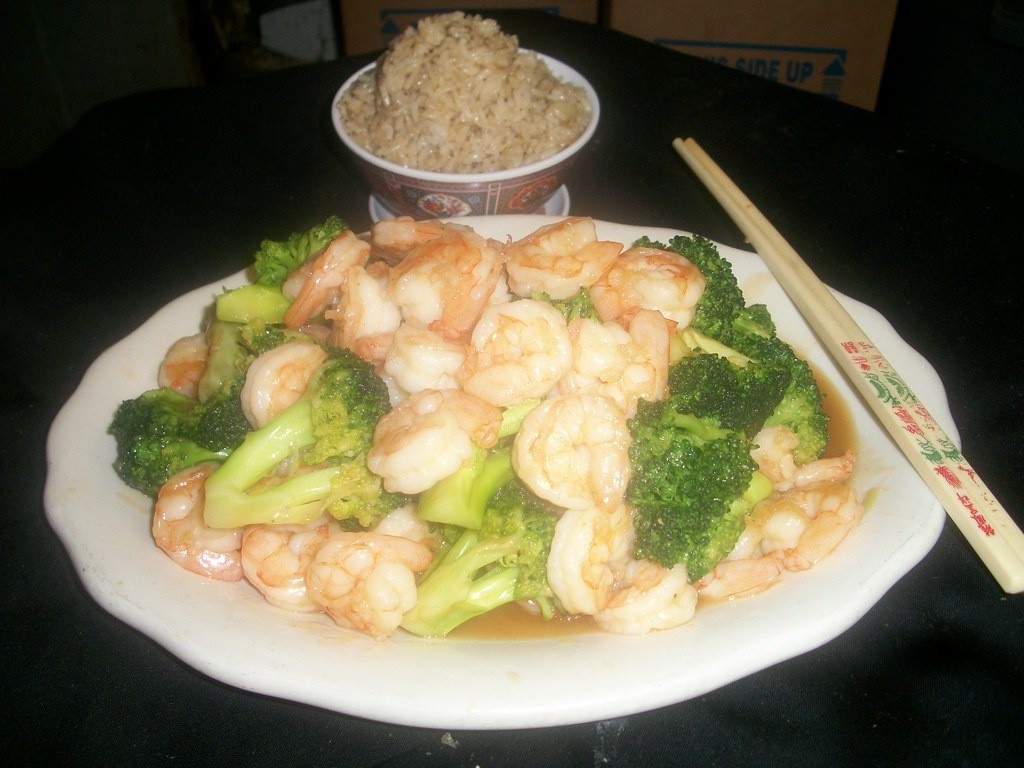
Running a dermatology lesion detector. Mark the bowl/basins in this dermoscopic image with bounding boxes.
[332,49,599,218]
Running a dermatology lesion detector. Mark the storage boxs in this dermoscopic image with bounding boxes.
[609,0,896,113]
[343,0,602,59]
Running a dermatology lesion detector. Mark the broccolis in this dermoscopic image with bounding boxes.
[106,213,831,639]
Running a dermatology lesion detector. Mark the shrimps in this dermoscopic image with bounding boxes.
[151,216,861,640]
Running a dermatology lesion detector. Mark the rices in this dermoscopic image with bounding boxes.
[336,10,588,176]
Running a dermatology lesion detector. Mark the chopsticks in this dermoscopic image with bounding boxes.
[671,137,1024,597]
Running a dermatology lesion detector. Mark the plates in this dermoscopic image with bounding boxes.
[40,214,964,729]
[368,183,570,224]
